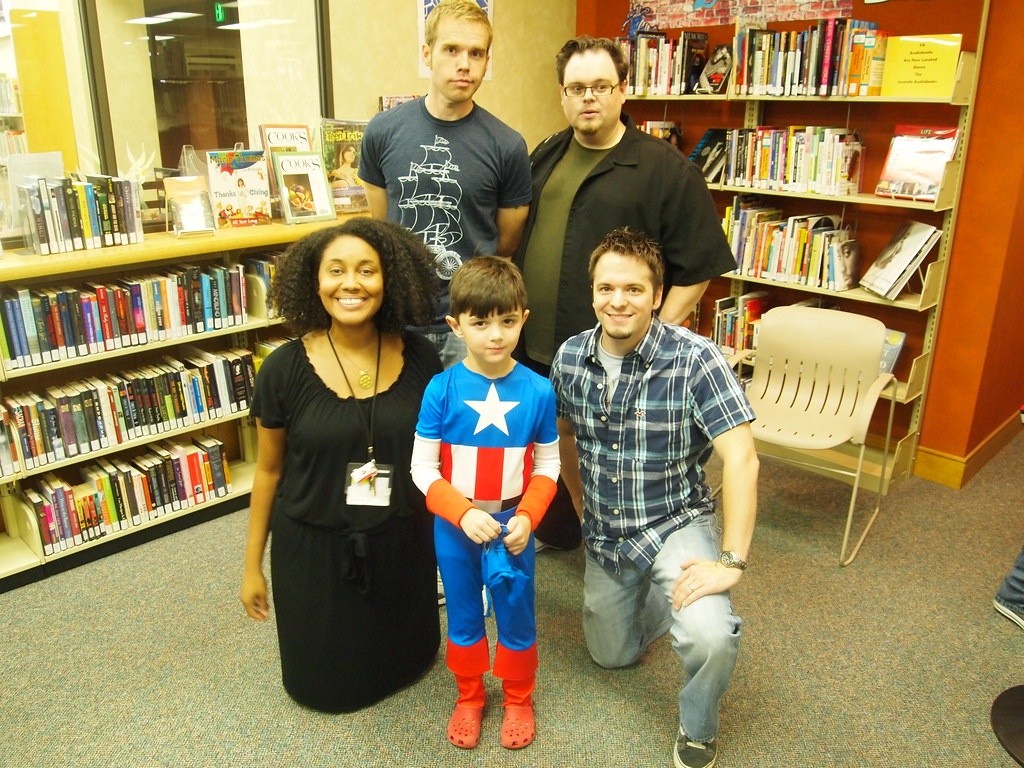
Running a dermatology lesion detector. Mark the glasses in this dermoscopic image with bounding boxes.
[561,78,626,97]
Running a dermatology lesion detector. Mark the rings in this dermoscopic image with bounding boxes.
[689,585,695,592]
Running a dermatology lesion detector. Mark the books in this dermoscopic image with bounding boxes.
[0,73,28,160]
[720,194,943,301]
[632,120,958,201]
[0,95,417,256]
[20,432,233,557]
[610,17,962,96]
[0,335,298,478]
[679,288,906,393]
[0,247,298,371]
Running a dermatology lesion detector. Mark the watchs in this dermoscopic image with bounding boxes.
[719,551,747,571]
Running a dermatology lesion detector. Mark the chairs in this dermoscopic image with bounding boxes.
[710,306,897,567]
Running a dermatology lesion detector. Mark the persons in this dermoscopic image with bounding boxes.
[329,144,358,187]
[353,1,535,370]
[836,241,860,290]
[409,254,562,749]
[239,216,446,716]
[699,45,731,91]
[513,37,739,551]
[993,544,1024,631]
[549,225,761,768]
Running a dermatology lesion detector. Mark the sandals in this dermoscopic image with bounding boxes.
[448,704,485,749]
[502,696,536,750]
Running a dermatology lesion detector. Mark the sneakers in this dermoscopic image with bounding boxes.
[673,721,719,768]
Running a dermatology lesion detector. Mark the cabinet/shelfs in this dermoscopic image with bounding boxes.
[623,0,992,498]
[0,8,80,230]
[0,213,373,594]
[185,80,249,150]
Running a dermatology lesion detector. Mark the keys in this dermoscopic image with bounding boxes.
[370,476,376,496]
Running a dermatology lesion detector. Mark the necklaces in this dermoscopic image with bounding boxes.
[334,344,377,389]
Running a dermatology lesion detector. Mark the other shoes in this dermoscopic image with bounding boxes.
[437,566,446,605]
[482,585,491,616]
[993,599,1024,633]
[535,535,582,552]
